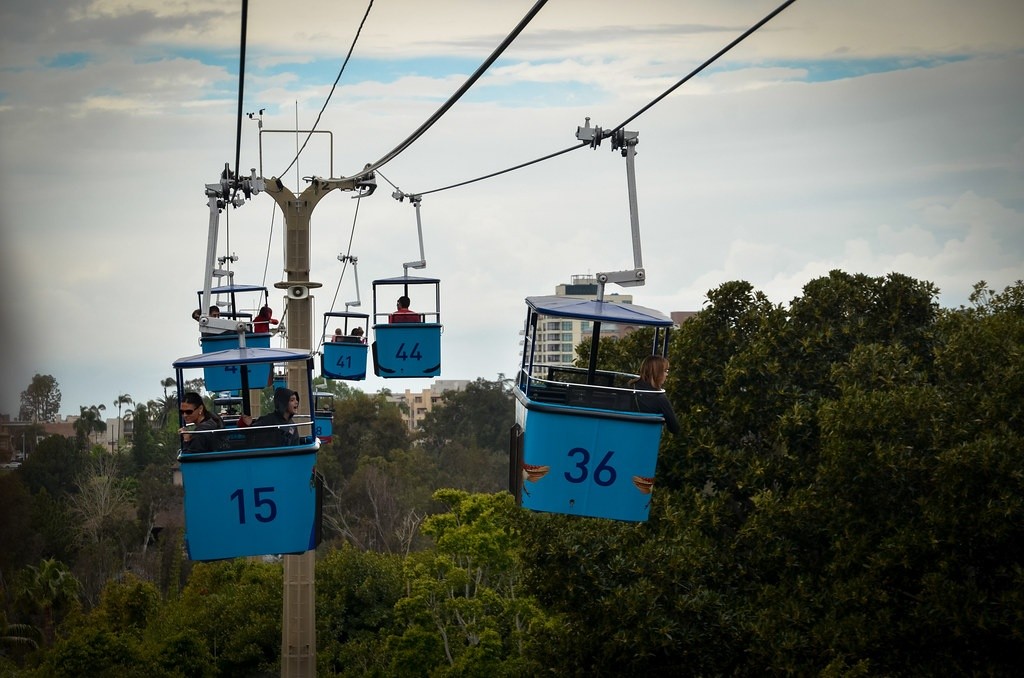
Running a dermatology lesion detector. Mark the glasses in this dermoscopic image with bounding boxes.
[179,406,199,414]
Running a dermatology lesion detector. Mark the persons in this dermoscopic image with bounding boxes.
[209,306,219,318]
[192,310,201,322]
[237,387,315,448]
[178,392,233,454]
[226,408,236,415]
[389,297,421,322]
[615,355,680,435]
[332,328,343,341]
[349,327,364,343]
[323,404,329,413]
[253,307,279,332]
